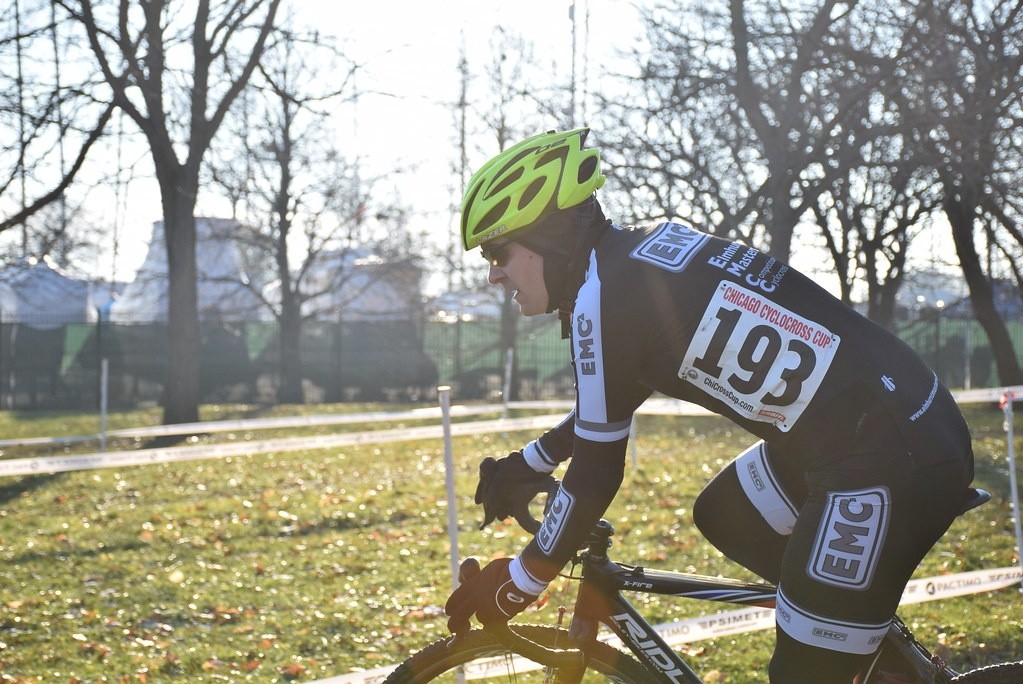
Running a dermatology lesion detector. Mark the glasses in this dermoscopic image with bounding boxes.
[480,235,521,267]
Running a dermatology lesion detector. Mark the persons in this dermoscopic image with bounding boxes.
[445,129,975,684]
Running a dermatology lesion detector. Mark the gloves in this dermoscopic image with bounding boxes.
[475,449,554,521]
[445,558,539,633]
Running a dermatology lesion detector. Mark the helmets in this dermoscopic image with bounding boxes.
[461,127,606,251]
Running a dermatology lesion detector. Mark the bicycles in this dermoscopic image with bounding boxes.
[383,469,1023,683]
